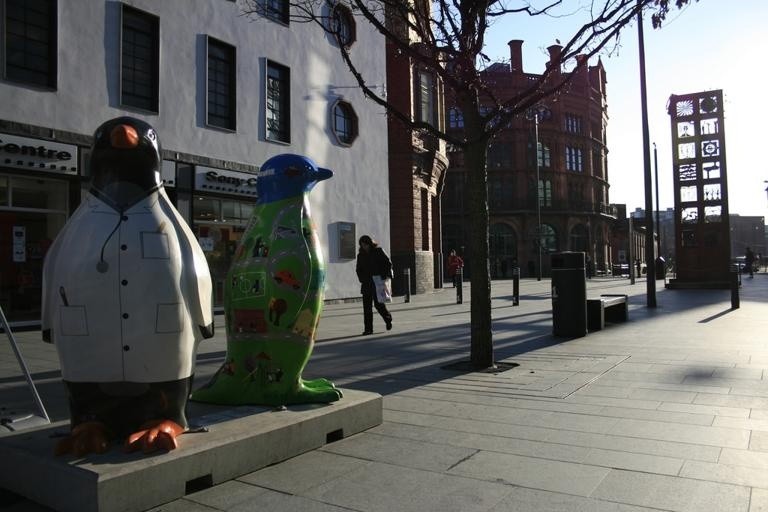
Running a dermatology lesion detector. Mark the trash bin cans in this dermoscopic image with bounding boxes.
[655,256,666,280]
[550,251,587,338]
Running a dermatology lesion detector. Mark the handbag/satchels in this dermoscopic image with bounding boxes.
[371,273,394,304]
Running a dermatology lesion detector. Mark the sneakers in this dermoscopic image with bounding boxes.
[362,330,374,335]
[385,320,393,331]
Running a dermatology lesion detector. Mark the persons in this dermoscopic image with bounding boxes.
[636,258,641,278]
[356,235,394,336]
[446,249,465,289]
[744,246,756,278]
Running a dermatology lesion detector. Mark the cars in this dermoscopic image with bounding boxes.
[733,255,746,272]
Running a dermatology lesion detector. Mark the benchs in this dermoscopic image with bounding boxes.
[585,294,630,331]
